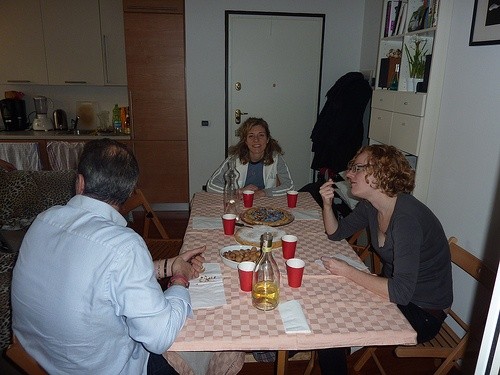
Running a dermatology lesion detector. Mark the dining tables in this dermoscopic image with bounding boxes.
[159,193,418,375]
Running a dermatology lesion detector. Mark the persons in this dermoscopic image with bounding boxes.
[207,117,293,198]
[319,144,453,375]
[12,138,206,375]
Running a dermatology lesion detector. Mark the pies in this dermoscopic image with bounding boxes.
[235,225,286,248]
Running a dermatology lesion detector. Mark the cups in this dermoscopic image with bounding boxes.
[97,110,109,133]
[285,258,306,288]
[242,190,254,208]
[287,190,298,208]
[281,235,298,258]
[5,90,23,98]
[221,213,237,236]
[237,261,257,292]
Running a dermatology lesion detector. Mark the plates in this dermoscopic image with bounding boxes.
[241,206,294,226]
[234,225,287,250]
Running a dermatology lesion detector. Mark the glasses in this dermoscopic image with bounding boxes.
[348,160,369,173]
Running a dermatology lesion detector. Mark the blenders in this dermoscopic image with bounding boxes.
[32,96,54,131]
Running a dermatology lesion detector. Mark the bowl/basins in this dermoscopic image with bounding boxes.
[219,245,262,269]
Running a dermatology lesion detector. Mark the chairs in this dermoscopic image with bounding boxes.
[349,218,497,375]
[118,187,183,261]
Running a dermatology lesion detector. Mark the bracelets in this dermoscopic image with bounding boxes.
[164,259,167,278]
[158,259,161,280]
[167,273,191,289]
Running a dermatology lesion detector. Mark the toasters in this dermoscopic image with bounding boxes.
[0,99,28,134]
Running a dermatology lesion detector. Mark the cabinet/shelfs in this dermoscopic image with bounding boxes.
[367,0,440,158]
[0,0,189,238]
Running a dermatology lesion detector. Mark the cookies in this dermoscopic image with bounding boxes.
[224,247,262,263]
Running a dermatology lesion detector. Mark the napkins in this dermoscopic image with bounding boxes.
[278,299,312,334]
[188,263,226,310]
[314,255,368,276]
[193,217,224,230]
[292,210,320,221]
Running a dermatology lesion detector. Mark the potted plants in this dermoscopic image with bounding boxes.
[404,38,425,91]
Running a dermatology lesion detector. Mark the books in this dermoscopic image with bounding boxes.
[385,1,400,37]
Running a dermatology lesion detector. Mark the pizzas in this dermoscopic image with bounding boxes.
[242,207,292,226]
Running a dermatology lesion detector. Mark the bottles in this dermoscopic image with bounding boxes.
[389,64,399,90]
[252,232,280,311]
[225,200,238,222]
[113,104,132,136]
[224,158,239,202]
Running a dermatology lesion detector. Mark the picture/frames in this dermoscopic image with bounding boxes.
[469,0,500,46]
